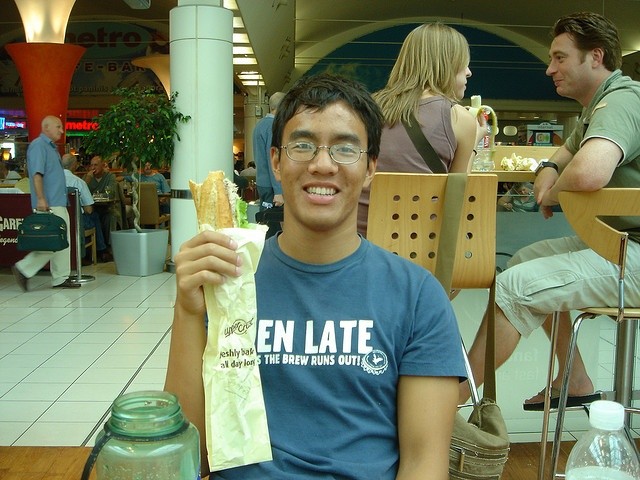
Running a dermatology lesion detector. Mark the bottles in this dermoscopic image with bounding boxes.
[472,113,495,172]
[564,399,640,479]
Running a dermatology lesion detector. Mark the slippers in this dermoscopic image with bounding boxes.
[522,385,601,411]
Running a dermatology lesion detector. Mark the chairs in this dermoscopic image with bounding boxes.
[241,179,257,200]
[66,185,97,264]
[364,171,499,413]
[538,186,640,480]
[136,182,170,243]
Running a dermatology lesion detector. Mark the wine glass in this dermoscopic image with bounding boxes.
[104,185,112,201]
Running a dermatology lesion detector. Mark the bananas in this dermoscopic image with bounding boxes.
[463,94,498,138]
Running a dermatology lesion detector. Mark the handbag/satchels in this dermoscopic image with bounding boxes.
[17,207,69,253]
[255,202,284,240]
[435,400,511,480]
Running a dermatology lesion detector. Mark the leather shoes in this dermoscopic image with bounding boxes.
[11,265,28,292]
[100,252,113,261]
[53,279,80,288]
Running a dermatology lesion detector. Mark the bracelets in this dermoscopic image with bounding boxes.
[473,149,477,156]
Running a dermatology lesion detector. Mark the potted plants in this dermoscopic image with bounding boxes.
[85,83,191,278]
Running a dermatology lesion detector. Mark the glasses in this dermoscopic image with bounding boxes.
[279,140,369,165]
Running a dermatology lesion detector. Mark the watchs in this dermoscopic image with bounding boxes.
[534,161,559,176]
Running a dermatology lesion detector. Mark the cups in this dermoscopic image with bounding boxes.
[81,390,201,480]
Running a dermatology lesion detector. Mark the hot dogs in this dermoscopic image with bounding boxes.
[188,170,248,229]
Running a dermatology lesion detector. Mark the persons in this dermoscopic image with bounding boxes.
[356,21,489,237]
[13,164,31,193]
[62,154,114,263]
[164,73,468,480]
[11,116,81,292]
[454,9,640,413]
[253,92,288,212]
[83,154,118,249]
[124,162,171,193]
[241,162,257,181]
